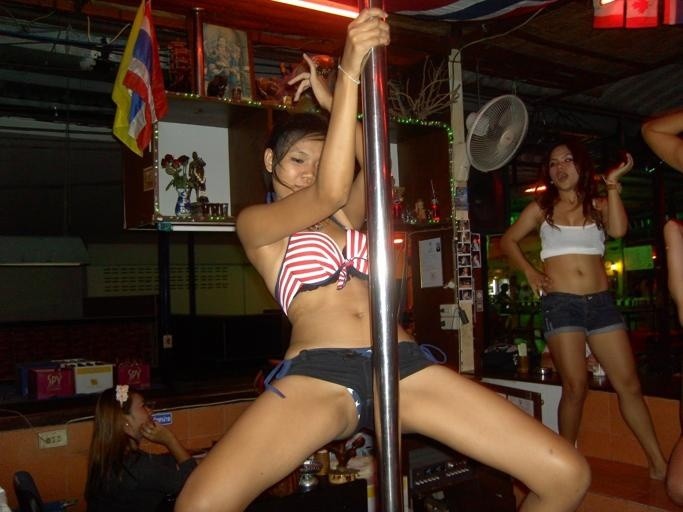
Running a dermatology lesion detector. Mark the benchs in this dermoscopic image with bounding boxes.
[513,390,680,512]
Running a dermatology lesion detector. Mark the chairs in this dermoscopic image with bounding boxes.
[14,470,78,512]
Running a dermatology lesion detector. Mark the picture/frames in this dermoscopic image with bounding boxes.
[191,21,256,101]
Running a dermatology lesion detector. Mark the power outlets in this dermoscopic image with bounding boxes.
[38,430,68,449]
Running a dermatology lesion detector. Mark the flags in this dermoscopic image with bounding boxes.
[123,0,171,151]
[111,0,144,159]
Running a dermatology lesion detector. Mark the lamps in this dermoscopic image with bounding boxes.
[0,0,92,266]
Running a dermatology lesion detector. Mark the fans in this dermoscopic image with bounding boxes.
[465,95,530,173]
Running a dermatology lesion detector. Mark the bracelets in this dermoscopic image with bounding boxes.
[606,183,624,193]
[335,62,362,92]
[337,64,360,86]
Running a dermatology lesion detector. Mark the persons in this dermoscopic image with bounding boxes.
[494,273,536,315]
[204,36,242,90]
[636,102,683,511]
[498,136,667,480]
[173,2,592,512]
[83,384,198,512]
